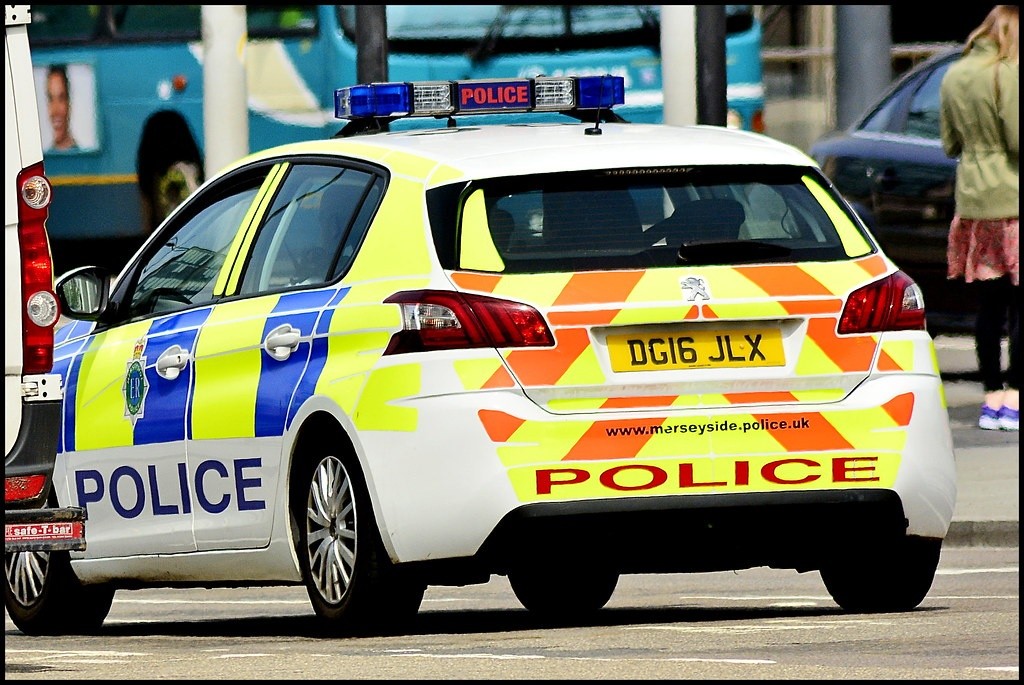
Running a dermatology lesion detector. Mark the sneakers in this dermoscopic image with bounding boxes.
[980,403,1019,431]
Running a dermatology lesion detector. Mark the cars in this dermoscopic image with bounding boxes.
[810,46,1012,333]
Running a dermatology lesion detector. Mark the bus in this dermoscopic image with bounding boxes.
[27,5,765,240]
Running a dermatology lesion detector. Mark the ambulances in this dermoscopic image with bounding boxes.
[1,74,957,636]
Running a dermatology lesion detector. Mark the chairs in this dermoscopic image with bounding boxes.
[664,198,744,245]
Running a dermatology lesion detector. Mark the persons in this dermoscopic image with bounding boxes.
[940,6,1019,432]
[45,63,81,153]
